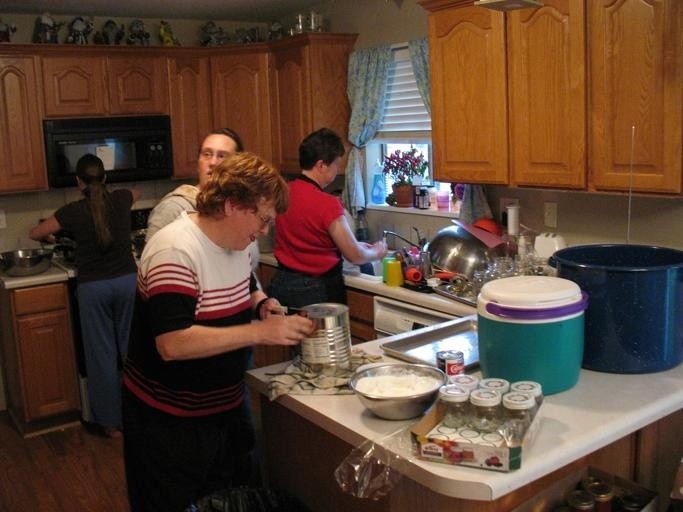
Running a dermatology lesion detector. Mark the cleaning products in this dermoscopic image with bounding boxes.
[371,158,385,204]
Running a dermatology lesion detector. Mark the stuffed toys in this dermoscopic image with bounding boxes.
[128,20,150,47]
[159,20,181,46]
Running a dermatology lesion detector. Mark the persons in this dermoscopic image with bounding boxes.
[120,144,316,512]
[30,153,142,436]
[94,19,125,45]
[146,129,243,243]
[66,17,96,45]
[268,127,390,361]
[32,12,66,43]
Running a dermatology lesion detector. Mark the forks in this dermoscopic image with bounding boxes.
[264,371,321,380]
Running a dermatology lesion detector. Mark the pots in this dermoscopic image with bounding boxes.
[131,226,148,257]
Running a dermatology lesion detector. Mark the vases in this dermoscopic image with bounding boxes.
[392,184,416,207]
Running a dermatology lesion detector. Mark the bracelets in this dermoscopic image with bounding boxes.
[256,298,269,320]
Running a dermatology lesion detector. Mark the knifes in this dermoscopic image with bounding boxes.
[271,304,309,318]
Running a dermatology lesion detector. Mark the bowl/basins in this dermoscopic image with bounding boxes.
[347,362,447,420]
[1,249,53,276]
[422,226,511,280]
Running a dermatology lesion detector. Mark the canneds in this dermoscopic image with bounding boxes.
[436,349,465,379]
[567,489,595,512]
[617,496,644,512]
[585,477,615,512]
[553,503,575,512]
[433,375,543,426]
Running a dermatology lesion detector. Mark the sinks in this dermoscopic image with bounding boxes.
[347,261,384,279]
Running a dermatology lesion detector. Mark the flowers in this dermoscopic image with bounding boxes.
[382,147,431,184]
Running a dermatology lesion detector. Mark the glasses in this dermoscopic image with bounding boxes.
[255,209,276,228]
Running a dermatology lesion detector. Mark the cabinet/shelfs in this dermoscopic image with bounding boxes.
[209,31,357,178]
[166,46,212,181]
[586,0,683,200]
[0,283,81,440]
[344,291,375,346]
[39,43,169,116]
[0,42,49,196]
[419,0,587,195]
[248,284,284,415]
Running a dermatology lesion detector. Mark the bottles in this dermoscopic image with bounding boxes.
[418,188,430,211]
[371,161,388,206]
[355,209,371,243]
[439,374,543,435]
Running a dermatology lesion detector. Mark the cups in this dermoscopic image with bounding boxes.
[472,254,554,297]
[437,191,449,210]
[382,257,397,283]
[408,246,420,268]
[420,251,432,278]
[407,267,421,281]
[387,262,405,287]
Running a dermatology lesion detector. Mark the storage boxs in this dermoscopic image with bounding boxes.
[588,464,661,512]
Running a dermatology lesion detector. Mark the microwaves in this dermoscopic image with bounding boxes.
[44,115,171,193]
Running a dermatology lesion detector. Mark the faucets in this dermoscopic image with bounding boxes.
[383,230,420,251]
